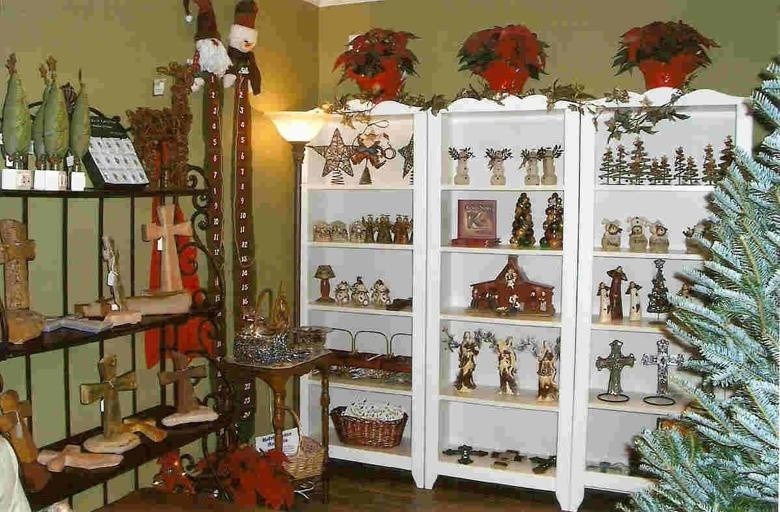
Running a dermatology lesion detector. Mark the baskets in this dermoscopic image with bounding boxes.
[249,406,325,482]
[312,328,412,387]
[330,406,408,447]
[235,289,288,365]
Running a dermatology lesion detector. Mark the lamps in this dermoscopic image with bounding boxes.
[264,109,332,430]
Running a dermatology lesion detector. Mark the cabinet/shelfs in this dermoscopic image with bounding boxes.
[572,86,754,512]
[295,103,425,489]
[427,96,581,512]
[2,102,240,510]
[329,20,727,87]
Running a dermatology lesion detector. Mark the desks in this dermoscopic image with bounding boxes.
[220,357,333,507]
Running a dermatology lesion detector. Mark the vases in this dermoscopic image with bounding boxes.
[476,60,529,94]
[635,55,703,91]
[346,56,407,103]
